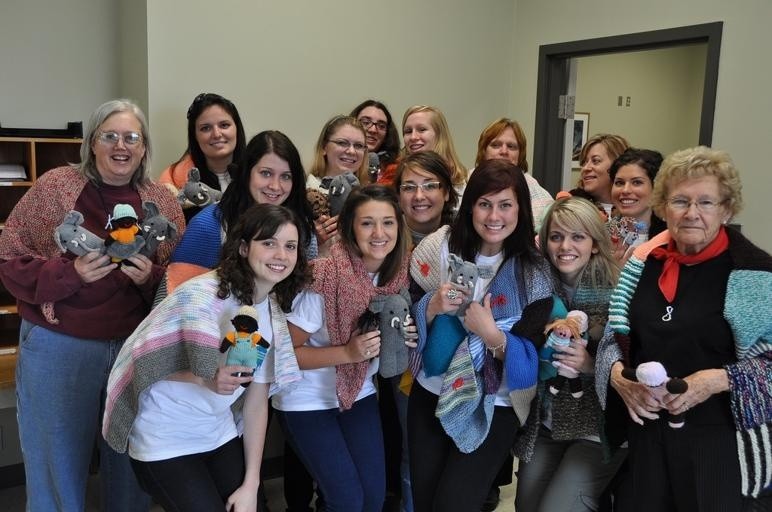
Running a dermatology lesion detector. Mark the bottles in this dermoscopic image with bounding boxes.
[106,203,138,230]
[231,304,260,328]
[635,361,666,387]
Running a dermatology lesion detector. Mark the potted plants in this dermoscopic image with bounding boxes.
[568,113,590,170]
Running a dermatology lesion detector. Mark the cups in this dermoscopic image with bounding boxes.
[91,131,146,145]
[398,181,442,193]
[664,195,731,211]
[359,118,389,131]
[327,138,369,150]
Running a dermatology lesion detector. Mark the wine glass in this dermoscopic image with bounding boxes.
[366,350,371,356]
[448,291,457,298]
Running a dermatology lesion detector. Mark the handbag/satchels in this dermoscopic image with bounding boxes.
[485,331,508,358]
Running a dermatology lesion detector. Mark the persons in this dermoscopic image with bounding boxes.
[399,105,468,210]
[393,151,460,303]
[594,146,772,512]
[103,203,313,512]
[516,198,634,511]
[409,159,555,511]
[349,99,400,191]
[459,117,555,249]
[154,130,318,324]
[304,115,370,259]
[272,187,419,512]
[605,146,666,270]
[0,99,187,512]
[570,134,630,221]
[160,92,246,225]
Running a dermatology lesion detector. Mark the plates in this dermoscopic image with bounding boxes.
[0,136,90,490]
